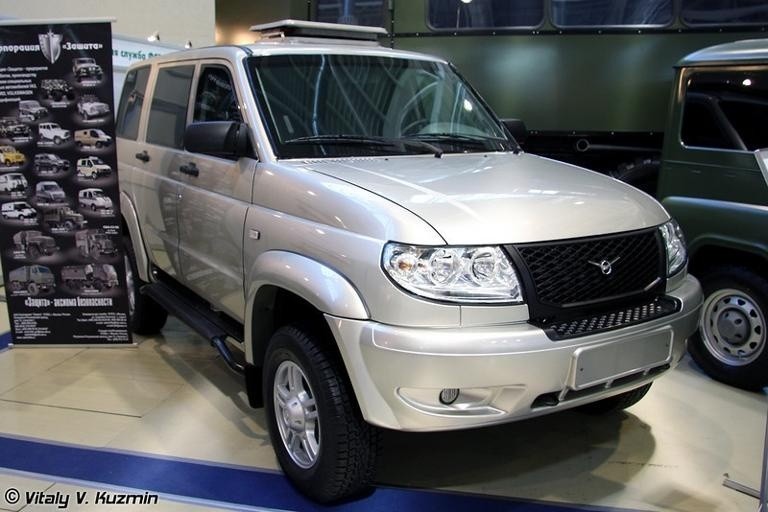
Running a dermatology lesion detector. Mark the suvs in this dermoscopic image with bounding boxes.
[0,57,121,295]
[114,19,704,505]
[659,35,768,383]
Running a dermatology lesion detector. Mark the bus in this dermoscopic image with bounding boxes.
[380,1,768,195]
